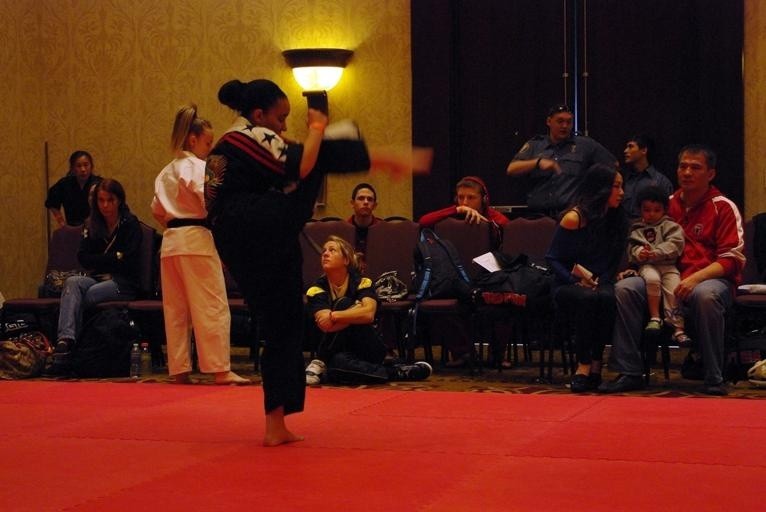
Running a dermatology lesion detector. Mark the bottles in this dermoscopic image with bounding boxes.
[130,343,141,379]
[139,343,152,380]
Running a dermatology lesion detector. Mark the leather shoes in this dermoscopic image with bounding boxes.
[590,370,601,394]
[571,364,590,393]
[705,380,729,396]
[599,377,645,393]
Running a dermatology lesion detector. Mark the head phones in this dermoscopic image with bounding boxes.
[454,176,491,206]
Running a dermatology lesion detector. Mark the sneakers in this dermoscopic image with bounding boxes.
[54,343,72,356]
[672,333,692,347]
[41,363,67,381]
[646,318,664,331]
[305,359,326,386]
[393,360,432,382]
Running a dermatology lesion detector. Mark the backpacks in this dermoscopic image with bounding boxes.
[1,330,54,381]
[412,228,471,296]
[474,249,554,293]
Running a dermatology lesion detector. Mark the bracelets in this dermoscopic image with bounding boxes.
[536,158,542,169]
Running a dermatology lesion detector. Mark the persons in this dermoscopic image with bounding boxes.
[603,143,748,395]
[418,175,511,370]
[626,182,693,346]
[621,132,675,232]
[44,150,104,228]
[148,101,254,385]
[52,177,144,360]
[305,235,434,388]
[504,103,621,219]
[346,182,385,228]
[545,161,631,393]
[203,76,436,449]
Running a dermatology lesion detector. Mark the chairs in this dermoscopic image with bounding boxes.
[0,212,766,384]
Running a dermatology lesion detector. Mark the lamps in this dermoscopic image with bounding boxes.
[280,47,354,93]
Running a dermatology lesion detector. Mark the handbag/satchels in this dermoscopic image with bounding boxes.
[1,312,36,341]
[45,270,90,298]
[374,271,408,303]
